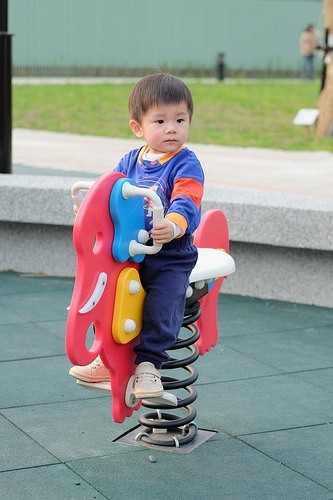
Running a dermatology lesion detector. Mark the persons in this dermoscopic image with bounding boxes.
[299,24,321,80]
[68,72,204,399]
[217,53,226,81]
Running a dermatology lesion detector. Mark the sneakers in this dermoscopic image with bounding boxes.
[69,356,110,382]
[133,362,163,398]
